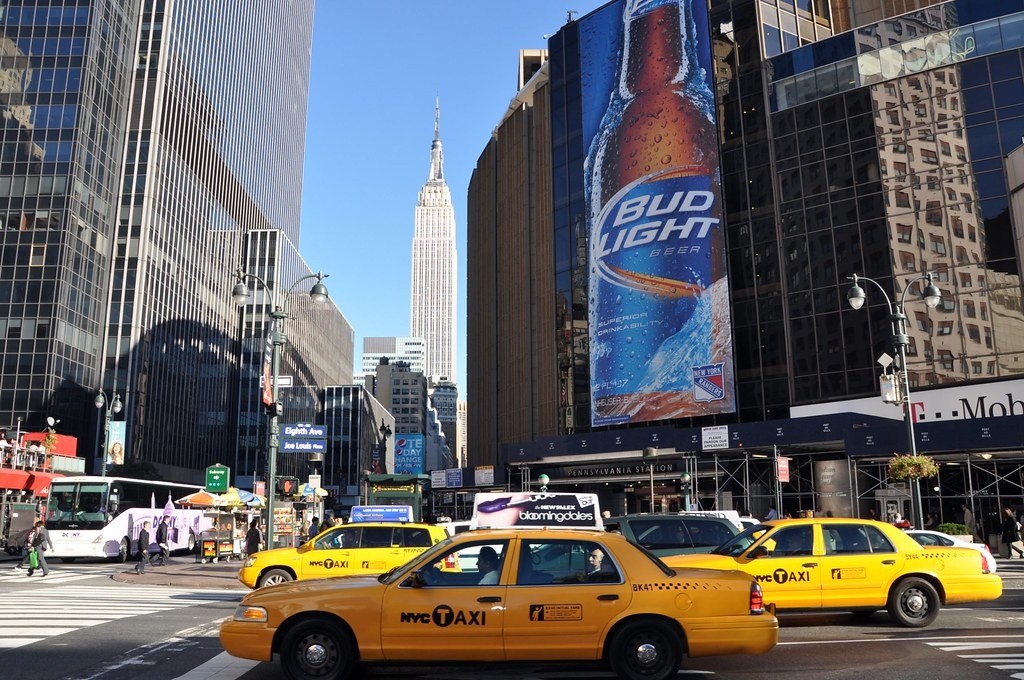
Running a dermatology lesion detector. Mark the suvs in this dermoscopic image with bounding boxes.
[435,509,768,572]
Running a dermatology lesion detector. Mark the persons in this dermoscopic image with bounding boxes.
[111,443,122,464]
[308,517,319,540]
[475,546,500,585]
[924,513,935,530]
[342,531,355,547]
[815,505,833,518]
[135,521,150,575]
[321,521,331,531]
[477,494,530,526]
[1001,508,1024,559]
[764,505,776,520]
[16,521,54,576]
[576,548,605,583]
[149,515,170,566]
[245,519,263,556]
[0,432,45,466]
[602,511,611,518]
[962,504,974,529]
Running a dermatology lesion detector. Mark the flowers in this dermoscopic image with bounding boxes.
[887,453,940,481]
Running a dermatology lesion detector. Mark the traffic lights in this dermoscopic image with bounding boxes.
[283,478,300,495]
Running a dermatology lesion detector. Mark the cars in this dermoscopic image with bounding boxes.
[237,504,463,589]
[218,492,780,680]
[904,530,998,573]
[657,517,1004,628]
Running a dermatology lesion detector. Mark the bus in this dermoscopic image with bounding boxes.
[34,476,220,564]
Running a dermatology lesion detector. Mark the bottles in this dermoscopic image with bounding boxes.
[584,0,745,427]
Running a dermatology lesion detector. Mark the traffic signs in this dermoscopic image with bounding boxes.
[260,376,293,388]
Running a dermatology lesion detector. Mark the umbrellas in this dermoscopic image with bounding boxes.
[293,483,328,497]
[174,486,267,507]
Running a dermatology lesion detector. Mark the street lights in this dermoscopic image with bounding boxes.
[231,266,331,551]
[845,272,943,530]
[94,387,123,475]
[378,418,393,474]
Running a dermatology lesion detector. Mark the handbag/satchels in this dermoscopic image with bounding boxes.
[1016,521,1022,531]
[32,527,45,547]
[30,547,39,569]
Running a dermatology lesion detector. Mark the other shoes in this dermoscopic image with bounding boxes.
[1006,554,1013,559]
[40,574,48,577]
[1020,552,1024,558]
[149,558,154,566]
[159,562,167,566]
[16,562,23,569]
[134,565,144,576]
[27,570,33,576]
